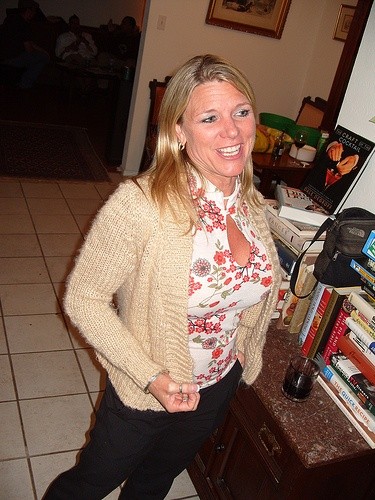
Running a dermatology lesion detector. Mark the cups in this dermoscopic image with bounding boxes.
[282,357,320,403]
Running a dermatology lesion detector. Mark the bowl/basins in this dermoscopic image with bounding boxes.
[289,142,316,162]
[259,112,321,146]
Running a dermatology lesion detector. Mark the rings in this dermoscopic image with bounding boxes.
[179,383,182,394]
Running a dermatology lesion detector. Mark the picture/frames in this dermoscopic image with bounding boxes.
[205,0,292,39]
[332,4,356,42]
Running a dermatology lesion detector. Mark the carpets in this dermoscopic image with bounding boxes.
[0,120,114,183]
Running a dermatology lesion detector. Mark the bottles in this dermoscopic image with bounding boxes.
[271,126,286,162]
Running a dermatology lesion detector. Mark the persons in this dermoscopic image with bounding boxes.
[44,54,282,500]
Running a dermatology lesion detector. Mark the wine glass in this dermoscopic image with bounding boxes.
[289,129,308,168]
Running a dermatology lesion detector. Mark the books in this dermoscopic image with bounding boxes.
[263,125,375,449]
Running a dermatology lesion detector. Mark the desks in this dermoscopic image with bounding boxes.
[50,61,119,104]
[252,152,315,199]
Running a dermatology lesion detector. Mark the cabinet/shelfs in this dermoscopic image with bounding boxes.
[187,327,375,500]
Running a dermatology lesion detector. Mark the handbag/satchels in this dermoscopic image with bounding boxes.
[289,207,375,299]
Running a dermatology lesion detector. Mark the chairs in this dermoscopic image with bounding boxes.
[296,96,327,129]
[139,76,172,174]
